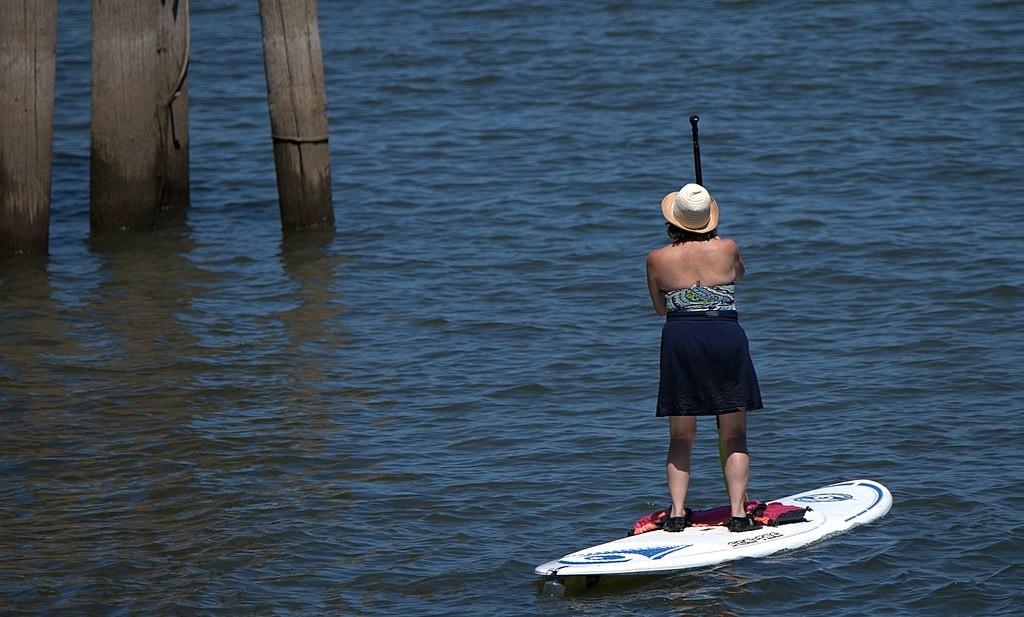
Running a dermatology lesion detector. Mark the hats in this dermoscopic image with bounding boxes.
[661,183,719,234]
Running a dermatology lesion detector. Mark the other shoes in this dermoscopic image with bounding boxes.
[663,516,684,532]
[729,515,762,532]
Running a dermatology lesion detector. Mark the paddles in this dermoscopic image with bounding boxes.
[690,114,748,505]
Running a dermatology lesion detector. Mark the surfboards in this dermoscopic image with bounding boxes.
[532,477,895,578]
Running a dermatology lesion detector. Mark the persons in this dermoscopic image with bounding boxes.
[646,184,763,532]
